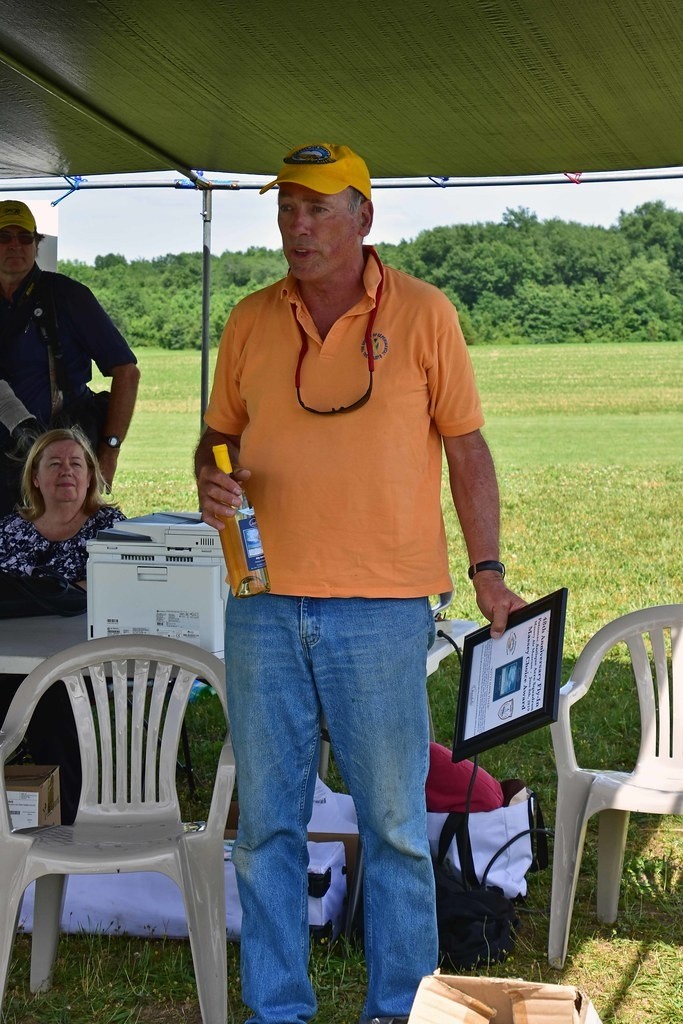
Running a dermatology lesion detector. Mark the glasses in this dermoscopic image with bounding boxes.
[0,231,37,244]
[295,366,373,416]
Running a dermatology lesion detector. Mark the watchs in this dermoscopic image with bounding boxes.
[100,435,121,449]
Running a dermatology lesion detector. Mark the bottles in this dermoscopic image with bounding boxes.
[212,443,271,598]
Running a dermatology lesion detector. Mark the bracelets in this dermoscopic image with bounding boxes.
[468,560,506,581]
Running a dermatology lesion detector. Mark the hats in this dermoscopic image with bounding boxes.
[258,143,371,203]
[0,199,36,234]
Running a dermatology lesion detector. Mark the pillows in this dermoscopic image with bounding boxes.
[426,741,505,814]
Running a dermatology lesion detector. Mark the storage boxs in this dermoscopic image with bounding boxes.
[406,975,603,1024]
[3,764,62,829]
[304,839,350,949]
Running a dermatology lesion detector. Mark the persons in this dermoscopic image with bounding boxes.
[194,143,528,1024]
[0,201,141,513]
[0,429,128,590]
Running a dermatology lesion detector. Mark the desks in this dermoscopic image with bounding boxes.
[0,607,483,953]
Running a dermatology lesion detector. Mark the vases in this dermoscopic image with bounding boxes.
[450,587,568,763]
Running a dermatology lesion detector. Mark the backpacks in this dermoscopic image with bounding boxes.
[429,814,515,973]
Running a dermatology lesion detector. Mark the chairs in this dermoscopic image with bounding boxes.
[0,630,237,1024]
[548,602,683,970]
